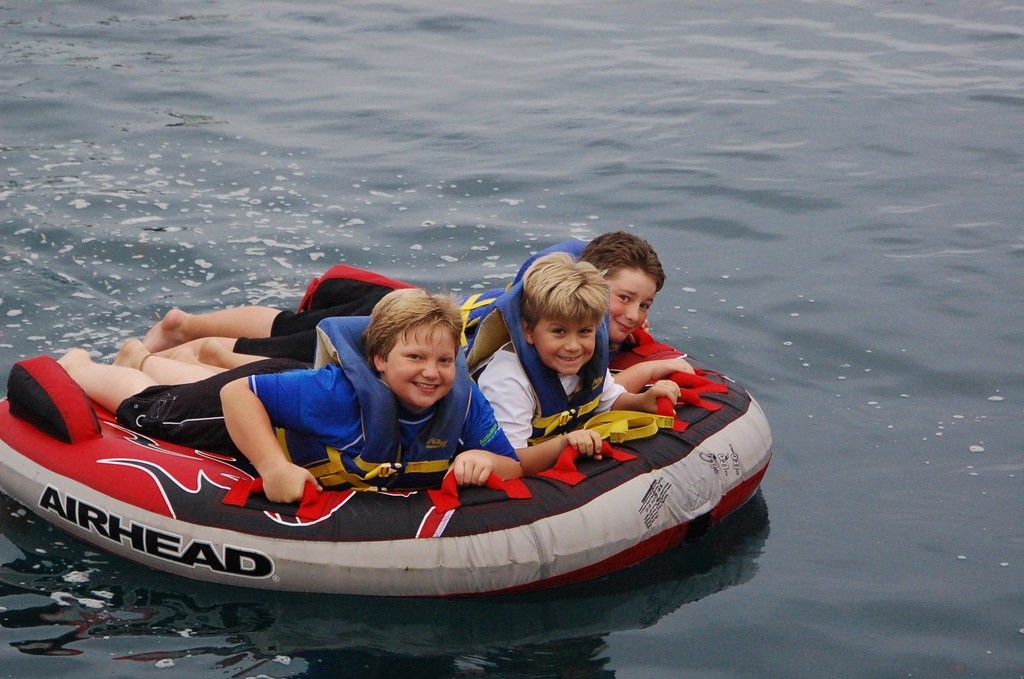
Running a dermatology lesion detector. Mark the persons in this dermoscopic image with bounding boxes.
[56,288,524,506]
[142,231,696,393]
[156,251,681,476]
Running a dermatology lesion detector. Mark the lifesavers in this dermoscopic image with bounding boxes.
[0,265,773,599]
